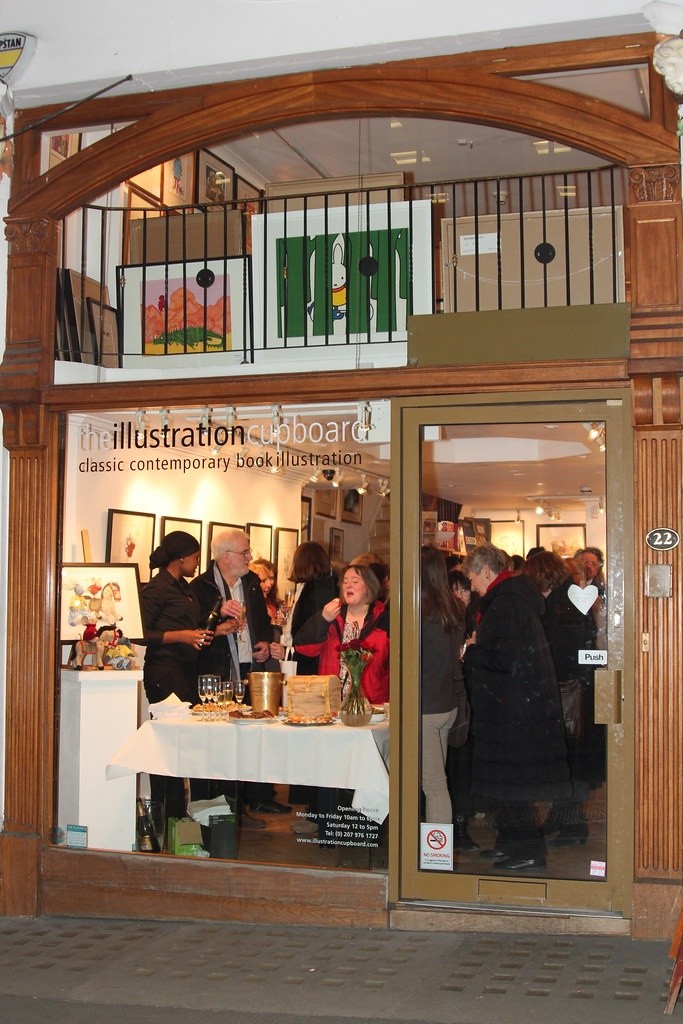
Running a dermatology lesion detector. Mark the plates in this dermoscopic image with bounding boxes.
[368,712,388,723]
[192,705,252,716]
[229,713,284,725]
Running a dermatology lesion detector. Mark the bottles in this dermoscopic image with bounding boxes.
[197,597,221,649]
[135,797,162,853]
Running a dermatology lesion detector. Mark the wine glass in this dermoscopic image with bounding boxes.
[234,600,246,643]
[198,674,245,722]
[282,588,296,622]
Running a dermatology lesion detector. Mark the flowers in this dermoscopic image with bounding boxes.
[336,621,376,684]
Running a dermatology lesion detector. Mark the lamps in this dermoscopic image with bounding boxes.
[582,419,609,452]
[535,497,562,520]
[598,494,606,512]
[357,474,371,494]
[331,466,343,487]
[137,406,291,474]
[515,510,522,522]
[311,460,323,482]
[377,479,391,496]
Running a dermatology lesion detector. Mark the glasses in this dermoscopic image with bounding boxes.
[225,547,252,558]
[452,588,470,596]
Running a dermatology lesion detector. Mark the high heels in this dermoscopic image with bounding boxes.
[549,823,589,848]
[538,816,563,835]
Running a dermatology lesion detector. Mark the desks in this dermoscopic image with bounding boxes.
[104,701,390,868]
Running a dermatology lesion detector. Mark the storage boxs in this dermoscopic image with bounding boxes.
[166,818,206,856]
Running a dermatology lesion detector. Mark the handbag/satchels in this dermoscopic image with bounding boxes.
[558,679,584,740]
[278,646,297,708]
[448,694,471,749]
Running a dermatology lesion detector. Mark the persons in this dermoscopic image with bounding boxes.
[421,542,604,870]
[128,530,390,835]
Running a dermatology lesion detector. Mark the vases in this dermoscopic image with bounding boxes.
[335,654,378,724]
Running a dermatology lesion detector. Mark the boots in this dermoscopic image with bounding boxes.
[454,816,480,849]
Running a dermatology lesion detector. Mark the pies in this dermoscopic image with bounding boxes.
[286,716,334,723]
[194,703,241,711]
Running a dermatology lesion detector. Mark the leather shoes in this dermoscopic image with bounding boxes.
[251,798,292,814]
[493,855,546,871]
[480,849,509,859]
[238,813,266,828]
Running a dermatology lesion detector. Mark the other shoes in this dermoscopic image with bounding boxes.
[288,791,314,805]
[290,820,319,835]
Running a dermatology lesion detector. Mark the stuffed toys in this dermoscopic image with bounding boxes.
[71,626,134,670]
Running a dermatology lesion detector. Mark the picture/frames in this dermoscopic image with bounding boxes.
[158,517,202,583]
[312,517,328,543]
[48,125,626,370]
[105,508,156,585]
[340,488,367,525]
[536,522,586,557]
[489,519,526,559]
[206,517,299,596]
[60,561,147,645]
[302,495,312,541]
[330,526,345,562]
[315,488,337,520]
